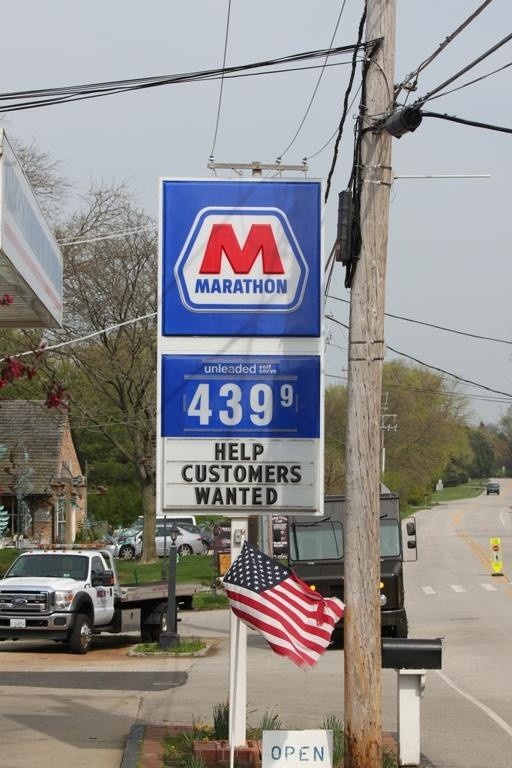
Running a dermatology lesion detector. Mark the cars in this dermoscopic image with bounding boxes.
[487,482,500,495]
[105,516,230,560]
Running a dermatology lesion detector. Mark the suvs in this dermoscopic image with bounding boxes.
[288,493,417,637]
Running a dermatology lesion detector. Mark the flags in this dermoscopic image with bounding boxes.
[222,544,346,675]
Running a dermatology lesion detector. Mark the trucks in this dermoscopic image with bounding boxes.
[0,549,196,654]
